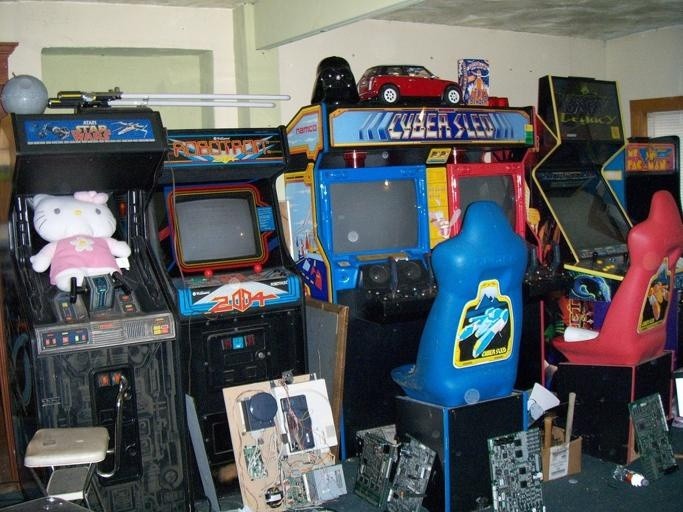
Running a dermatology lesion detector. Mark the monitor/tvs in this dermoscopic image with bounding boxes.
[447,163,526,245]
[165,186,264,274]
[317,166,430,261]
[533,169,634,255]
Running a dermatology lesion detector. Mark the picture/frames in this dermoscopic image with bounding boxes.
[305,296,349,462]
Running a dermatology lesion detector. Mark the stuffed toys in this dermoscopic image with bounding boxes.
[28,188,132,292]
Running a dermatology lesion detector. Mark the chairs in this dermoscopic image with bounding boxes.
[390,201,527,406]
[24,375,131,512]
[552,191,683,366]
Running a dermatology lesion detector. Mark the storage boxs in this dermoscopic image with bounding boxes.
[539,426,582,483]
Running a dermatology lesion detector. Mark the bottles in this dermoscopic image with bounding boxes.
[612,465,649,488]
